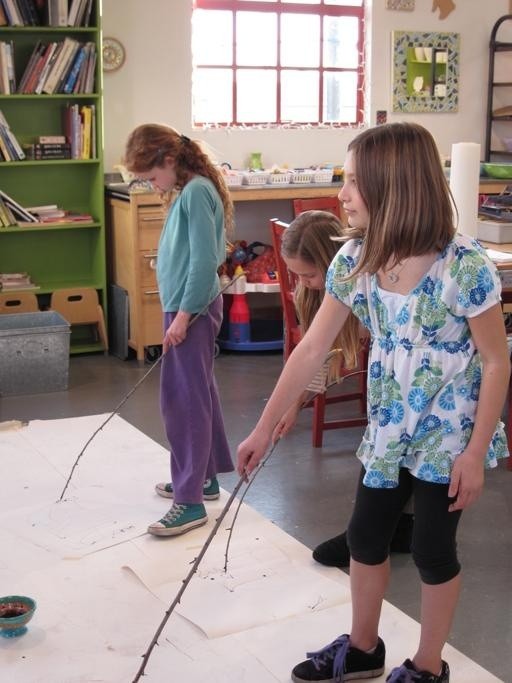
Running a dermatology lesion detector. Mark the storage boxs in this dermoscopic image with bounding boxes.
[1,310,72,399]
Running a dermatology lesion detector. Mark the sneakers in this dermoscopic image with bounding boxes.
[391,513,412,551]
[291,634,385,683]
[147,503,206,535]
[313,530,350,566]
[156,477,219,500]
[387,656,450,683]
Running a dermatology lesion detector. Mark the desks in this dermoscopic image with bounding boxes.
[100,171,342,364]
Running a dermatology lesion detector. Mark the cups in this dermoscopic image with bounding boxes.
[414,46,432,62]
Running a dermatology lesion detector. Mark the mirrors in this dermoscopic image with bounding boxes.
[393,32,459,110]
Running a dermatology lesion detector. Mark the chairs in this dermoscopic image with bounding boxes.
[269,196,371,449]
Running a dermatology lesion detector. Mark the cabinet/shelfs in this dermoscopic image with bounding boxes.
[486,16,511,165]
[1,1,108,357]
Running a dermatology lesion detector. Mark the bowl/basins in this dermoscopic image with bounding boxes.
[482,162,512,179]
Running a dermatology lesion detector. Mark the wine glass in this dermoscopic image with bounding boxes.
[0,593,38,639]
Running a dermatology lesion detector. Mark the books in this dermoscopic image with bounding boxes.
[0,0,102,291]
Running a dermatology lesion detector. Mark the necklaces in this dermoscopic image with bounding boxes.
[387,257,409,285]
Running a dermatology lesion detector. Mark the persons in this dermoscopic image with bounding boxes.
[123,121,239,537]
[235,120,510,682]
[270,209,417,570]
